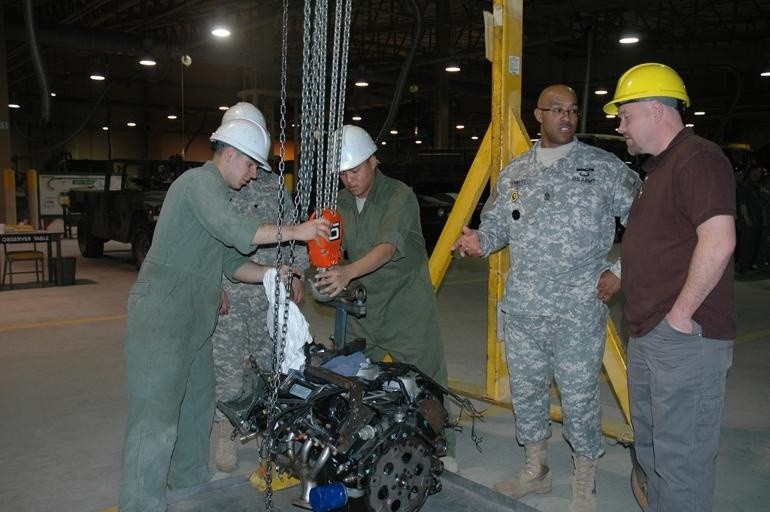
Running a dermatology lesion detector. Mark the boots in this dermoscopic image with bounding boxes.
[570,453,597,511]
[215,419,240,472]
[495,440,552,497]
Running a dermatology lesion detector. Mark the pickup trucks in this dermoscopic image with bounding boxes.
[64,157,211,276]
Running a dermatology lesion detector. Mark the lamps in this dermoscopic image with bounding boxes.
[445,62,461,72]
[355,77,368,86]
[139,37,157,66]
[619,24,639,44]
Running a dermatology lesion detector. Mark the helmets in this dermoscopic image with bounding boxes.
[332,124,377,172]
[222,102,267,128]
[603,62,691,114]
[210,119,272,172]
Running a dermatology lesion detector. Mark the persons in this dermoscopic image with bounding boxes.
[450,85,644,508]
[117,120,332,510]
[730,163,770,278]
[601,61,740,511]
[311,122,448,424]
[209,101,309,477]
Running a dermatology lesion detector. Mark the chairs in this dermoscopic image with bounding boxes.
[3,240,45,288]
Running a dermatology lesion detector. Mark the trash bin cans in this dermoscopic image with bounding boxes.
[52,256,77,286]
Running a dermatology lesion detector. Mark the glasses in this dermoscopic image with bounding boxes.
[541,108,583,118]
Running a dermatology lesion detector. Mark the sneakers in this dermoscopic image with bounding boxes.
[210,471,230,480]
[440,456,459,473]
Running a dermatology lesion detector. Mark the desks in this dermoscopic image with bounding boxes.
[0,233,67,286]
